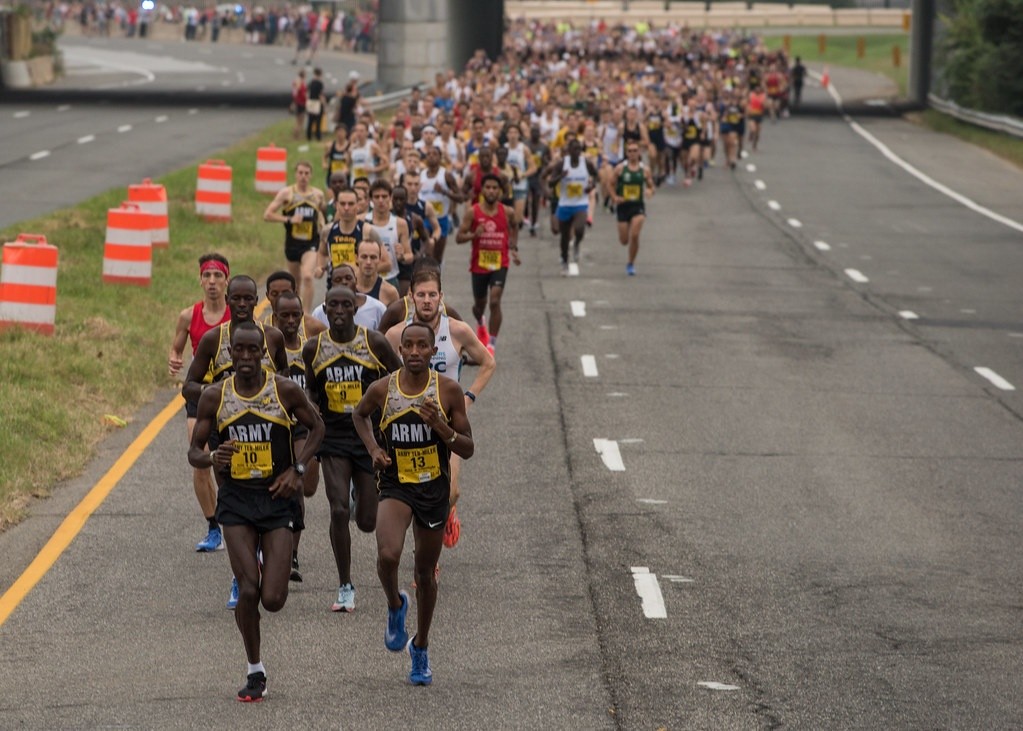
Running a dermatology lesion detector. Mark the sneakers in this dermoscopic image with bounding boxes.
[478,317,488,344]
[196,529,223,551]
[486,334,495,358]
[226,579,239,610]
[331,582,356,613]
[383,589,410,653]
[442,504,461,547]
[412,566,440,589]
[406,637,433,686]
[238,671,267,702]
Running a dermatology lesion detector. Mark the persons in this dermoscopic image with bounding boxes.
[385,271,496,589]
[182,5,378,64]
[169,253,231,551]
[354,323,474,685]
[183,275,290,609]
[188,322,327,702]
[263,16,808,358]
[302,285,402,612]
[274,291,320,581]
[260,270,327,342]
[49,0,149,37]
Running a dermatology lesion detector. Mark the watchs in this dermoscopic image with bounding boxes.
[292,463,305,475]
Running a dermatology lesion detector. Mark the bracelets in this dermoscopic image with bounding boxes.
[511,245,518,251]
[465,391,475,402]
[201,384,205,393]
[288,216,291,223]
[445,431,457,444]
[210,451,215,464]
[431,237,436,242]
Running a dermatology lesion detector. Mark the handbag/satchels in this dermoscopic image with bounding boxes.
[305,99,321,114]
[289,103,298,115]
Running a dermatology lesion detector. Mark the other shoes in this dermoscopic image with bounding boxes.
[289,562,302,584]
[571,241,580,262]
[627,263,634,275]
[560,257,568,276]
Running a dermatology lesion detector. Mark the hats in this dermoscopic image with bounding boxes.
[348,72,359,80]
